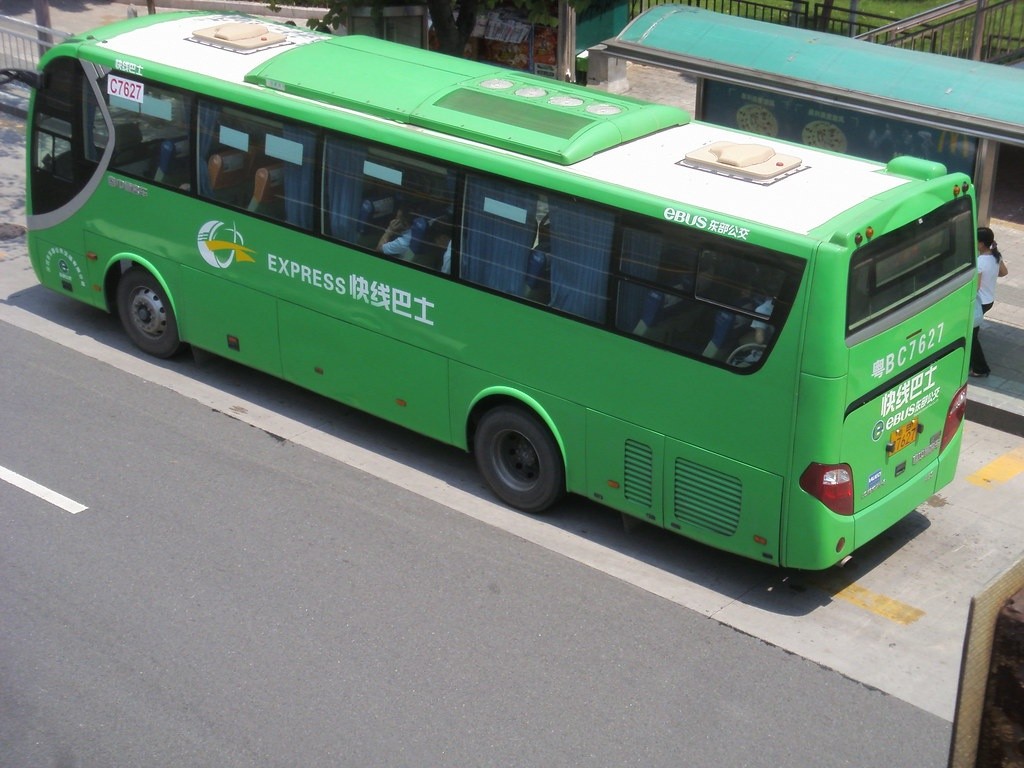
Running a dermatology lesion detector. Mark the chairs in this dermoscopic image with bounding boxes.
[633,282,760,361]
[152,134,287,218]
[361,194,456,264]
[522,241,574,303]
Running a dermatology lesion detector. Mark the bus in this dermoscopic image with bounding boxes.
[1,10,981,579]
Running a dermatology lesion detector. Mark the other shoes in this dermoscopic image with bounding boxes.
[968,370,989,377]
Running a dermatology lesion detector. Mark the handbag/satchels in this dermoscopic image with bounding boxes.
[973,298,983,328]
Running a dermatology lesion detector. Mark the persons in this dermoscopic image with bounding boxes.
[377,205,455,274]
[750,271,778,345]
[969,227,1008,377]
